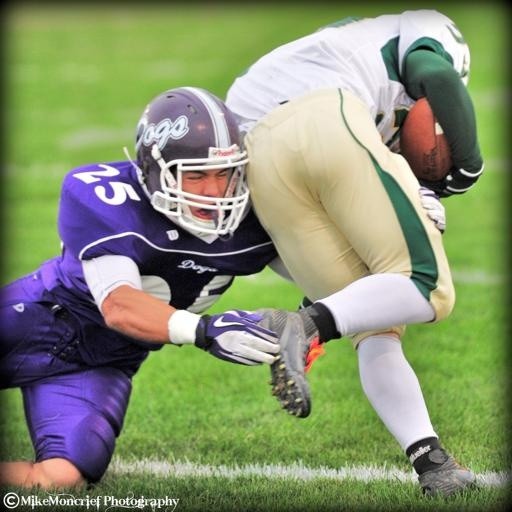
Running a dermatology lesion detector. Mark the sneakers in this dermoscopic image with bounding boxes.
[417,458,486,500]
[267,306,318,417]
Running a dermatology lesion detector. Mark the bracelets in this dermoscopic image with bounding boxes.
[168,310,201,345]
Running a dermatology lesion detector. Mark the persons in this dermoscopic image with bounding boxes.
[226,9,512,502]
[0,86,447,492]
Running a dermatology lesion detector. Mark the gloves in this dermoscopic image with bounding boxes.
[194,309,280,365]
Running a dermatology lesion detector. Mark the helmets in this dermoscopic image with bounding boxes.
[135,88,250,234]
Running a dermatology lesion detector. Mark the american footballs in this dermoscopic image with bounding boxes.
[400,97,453,183]
